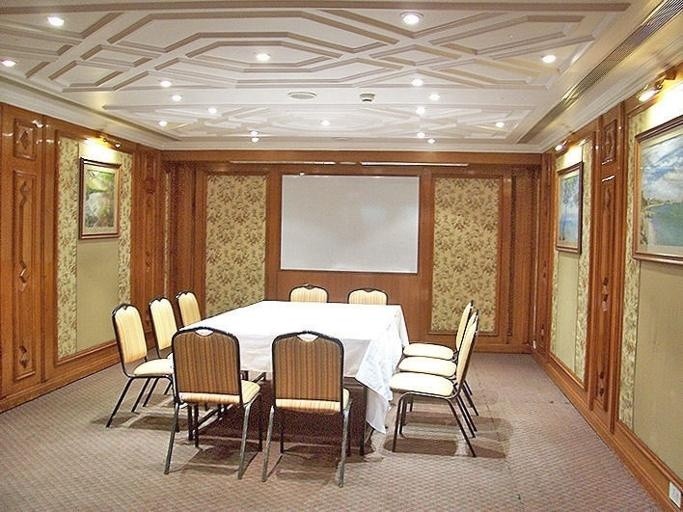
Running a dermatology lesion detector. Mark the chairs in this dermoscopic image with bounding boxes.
[163,327,263,480]
[148,295,180,359]
[389,315,479,458]
[288,283,329,304]
[175,290,201,328]
[106,303,210,441]
[397,309,479,432]
[261,329,353,488]
[347,287,387,306]
[401,300,475,408]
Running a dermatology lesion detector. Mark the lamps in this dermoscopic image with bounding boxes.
[636,67,676,102]
[554,138,568,151]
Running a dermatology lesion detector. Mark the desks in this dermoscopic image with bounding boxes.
[175,298,409,457]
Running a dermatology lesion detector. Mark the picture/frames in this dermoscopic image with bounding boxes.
[78,157,122,240]
[631,115,683,265]
[554,160,584,255]
